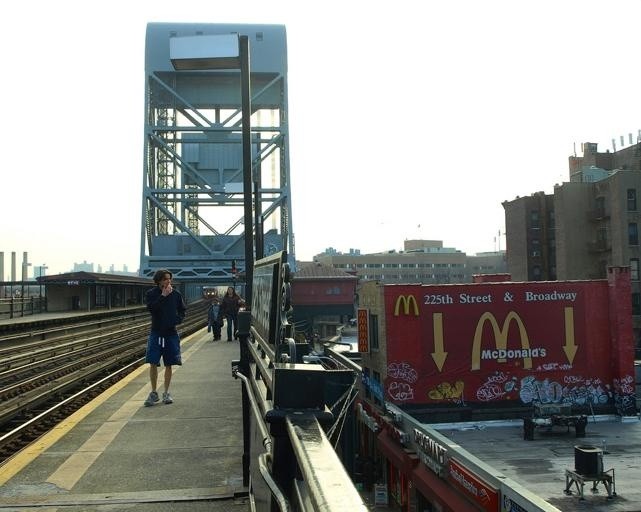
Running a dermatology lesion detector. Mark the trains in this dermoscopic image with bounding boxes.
[202,288,216,299]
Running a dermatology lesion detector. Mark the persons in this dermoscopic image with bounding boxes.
[221,286,245,342]
[207,298,224,341]
[144,269,189,406]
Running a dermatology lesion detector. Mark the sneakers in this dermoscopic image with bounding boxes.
[144,391,160,406]
[162,392,174,404]
